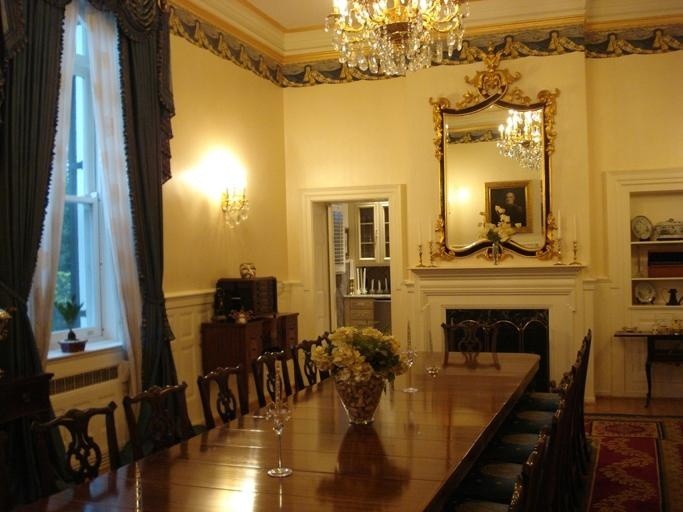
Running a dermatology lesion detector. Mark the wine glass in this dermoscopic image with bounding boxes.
[403,350,420,395]
[266,409,295,479]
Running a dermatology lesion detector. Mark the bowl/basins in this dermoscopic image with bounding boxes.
[655,218,683,239]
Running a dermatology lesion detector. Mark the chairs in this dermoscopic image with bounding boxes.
[19,398,121,496]
[435,325,593,512]
[439,317,501,355]
[251,347,292,407]
[289,330,338,392]
[197,362,251,429]
[120,381,194,460]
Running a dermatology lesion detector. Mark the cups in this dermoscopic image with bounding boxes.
[426,367,440,379]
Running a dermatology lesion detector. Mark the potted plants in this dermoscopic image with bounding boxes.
[55,297,88,353]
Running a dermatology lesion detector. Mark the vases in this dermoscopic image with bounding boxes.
[491,242,499,266]
[333,371,386,426]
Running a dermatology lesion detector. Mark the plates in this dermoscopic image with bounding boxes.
[631,215,653,239]
[634,281,656,303]
[622,326,638,332]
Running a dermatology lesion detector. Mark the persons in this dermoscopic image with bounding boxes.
[501,193,523,226]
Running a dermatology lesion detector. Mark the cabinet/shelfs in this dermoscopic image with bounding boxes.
[215,276,278,318]
[625,186,683,310]
[342,295,375,329]
[346,201,390,269]
[196,313,301,414]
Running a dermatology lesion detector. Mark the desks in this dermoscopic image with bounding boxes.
[3,350,543,512]
[610,326,682,411]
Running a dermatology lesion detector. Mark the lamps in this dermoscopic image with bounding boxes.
[324,0,471,76]
[495,107,544,170]
[220,187,248,230]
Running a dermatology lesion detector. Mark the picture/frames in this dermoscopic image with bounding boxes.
[483,180,533,235]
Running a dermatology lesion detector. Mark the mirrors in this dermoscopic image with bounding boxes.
[425,37,560,262]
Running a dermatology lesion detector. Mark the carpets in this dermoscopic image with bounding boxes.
[576,412,682,511]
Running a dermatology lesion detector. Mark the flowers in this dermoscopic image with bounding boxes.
[308,322,408,387]
[476,205,521,245]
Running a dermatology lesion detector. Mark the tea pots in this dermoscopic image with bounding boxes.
[659,288,683,306]
